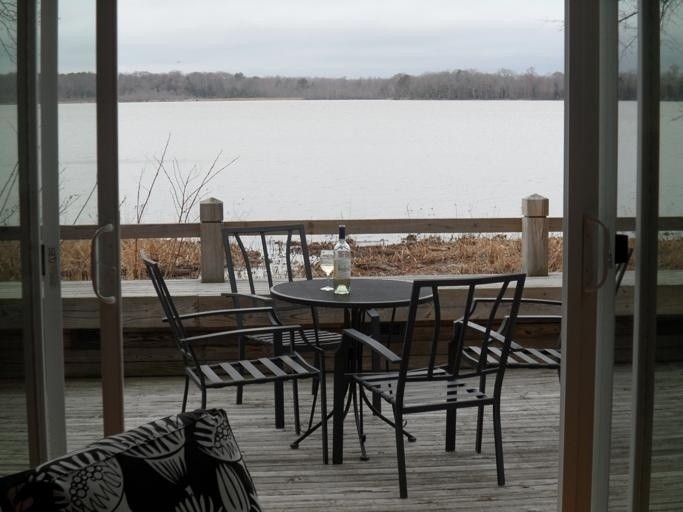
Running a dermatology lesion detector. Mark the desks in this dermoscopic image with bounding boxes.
[269,280,434,461]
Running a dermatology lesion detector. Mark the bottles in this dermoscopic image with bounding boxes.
[333,224,351,294]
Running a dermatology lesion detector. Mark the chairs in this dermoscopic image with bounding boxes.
[221,225,382,430]
[342,272,528,499]
[140,244,329,466]
[452,233,634,452]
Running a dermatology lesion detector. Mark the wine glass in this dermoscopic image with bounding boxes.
[319,250,335,292]
